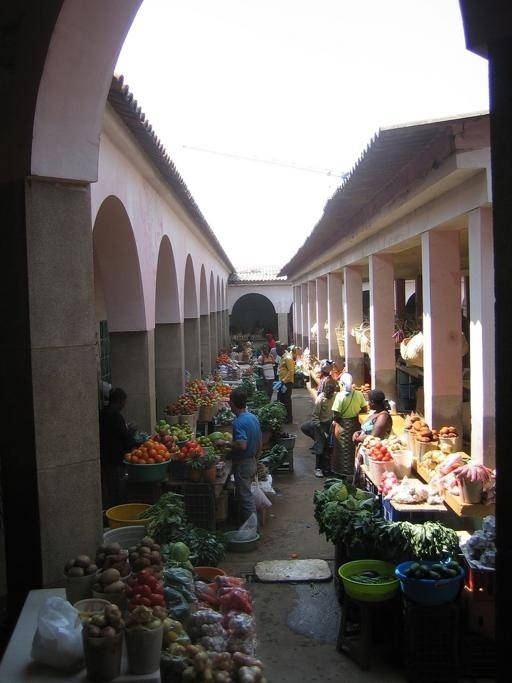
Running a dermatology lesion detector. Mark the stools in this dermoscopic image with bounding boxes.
[400,592,459,683]
[335,593,399,671]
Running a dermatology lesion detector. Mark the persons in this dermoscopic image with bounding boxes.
[212,386,264,531]
[265,332,276,349]
[351,387,393,490]
[328,372,368,483]
[274,343,296,424]
[269,340,281,382]
[300,379,340,455]
[98,386,143,510]
[316,357,337,400]
[253,342,277,399]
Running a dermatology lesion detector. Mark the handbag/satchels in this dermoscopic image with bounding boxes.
[327,420,336,447]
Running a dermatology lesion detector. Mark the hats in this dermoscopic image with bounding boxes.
[320,359,335,374]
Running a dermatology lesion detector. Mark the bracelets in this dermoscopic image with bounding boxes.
[224,439,230,448]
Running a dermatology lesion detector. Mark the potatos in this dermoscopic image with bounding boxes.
[404,416,458,442]
[63,536,165,602]
[80,602,269,683]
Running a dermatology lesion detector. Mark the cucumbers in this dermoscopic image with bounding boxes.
[405,561,460,580]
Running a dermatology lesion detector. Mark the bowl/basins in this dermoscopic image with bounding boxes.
[123,434,188,483]
[223,530,259,552]
[102,502,156,550]
[337,559,400,603]
[270,435,296,450]
[395,558,466,604]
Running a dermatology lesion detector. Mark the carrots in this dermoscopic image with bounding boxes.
[195,575,252,613]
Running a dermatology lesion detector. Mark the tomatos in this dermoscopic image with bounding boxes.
[367,442,392,462]
[123,438,206,463]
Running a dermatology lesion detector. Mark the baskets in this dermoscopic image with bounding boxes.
[183,484,216,531]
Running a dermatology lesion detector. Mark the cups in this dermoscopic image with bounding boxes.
[61,567,163,683]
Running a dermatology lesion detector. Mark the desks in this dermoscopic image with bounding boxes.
[0,589,161,683]
[153,353,259,530]
[296,354,470,532]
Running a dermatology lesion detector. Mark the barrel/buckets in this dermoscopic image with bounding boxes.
[103,526,148,550]
[106,503,154,527]
[361,430,464,484]
[458,478,483,504]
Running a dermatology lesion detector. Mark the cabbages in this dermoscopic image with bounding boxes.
[207,431,233,441]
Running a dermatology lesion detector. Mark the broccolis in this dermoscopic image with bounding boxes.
[327,484,366,501]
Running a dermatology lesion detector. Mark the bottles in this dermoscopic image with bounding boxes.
[272,382,282,391]
